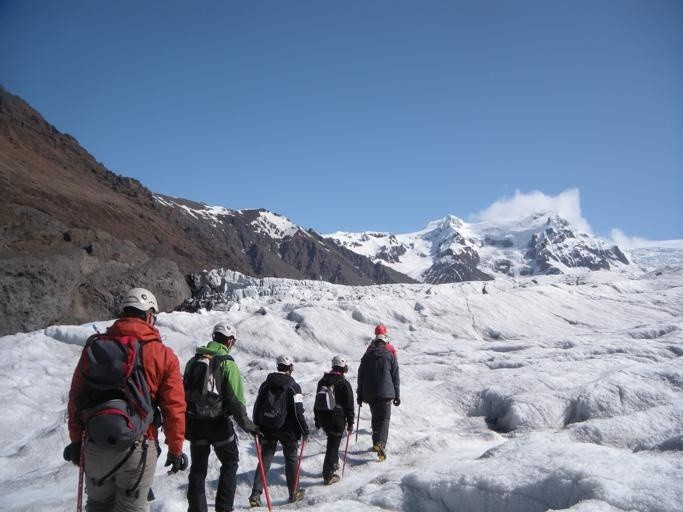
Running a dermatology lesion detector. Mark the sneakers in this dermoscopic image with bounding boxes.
[333,463,339,471]
[288,489,304,504]
[372,440,386,461]
[248,494,262,507]
[324,473,339,485]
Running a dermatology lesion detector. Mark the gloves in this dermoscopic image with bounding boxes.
[357,398,362,407]
[63,440,80,465]
[251,426,264,438]
[347,423,353,434]
[314,417,320,429]
[393,398,400,406]
[165,453,187,475]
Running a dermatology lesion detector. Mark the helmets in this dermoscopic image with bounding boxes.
[331,355,347,367]
[374,324,386,334]
[211,323,237,340]
[374,335,389,343]
[119,287,158,313]
[276,354,293,366]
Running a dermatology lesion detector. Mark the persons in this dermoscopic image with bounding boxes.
[63,287,187,512]
[356,324,401,461]
[247,354,308,507]
[184,321,265,512]
[313,355,354,485]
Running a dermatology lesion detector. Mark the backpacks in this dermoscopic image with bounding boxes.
[74,334,154,451]
[253,373,290,431]
[314,375,345,411]
[182,353,234,421]
